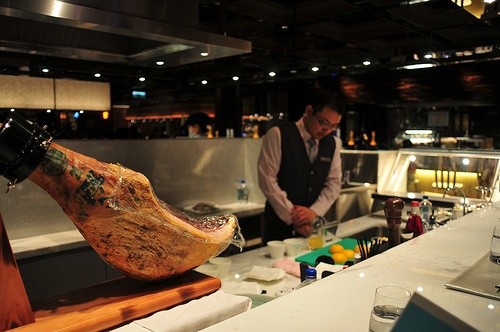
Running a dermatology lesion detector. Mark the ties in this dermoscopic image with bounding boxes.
[308,138,317,164]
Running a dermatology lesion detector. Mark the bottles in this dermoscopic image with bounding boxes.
[426,215,440,232]
[237,179,247,202]
[347,129,377,150]
[404,201,424,239]
[419,196,432,229]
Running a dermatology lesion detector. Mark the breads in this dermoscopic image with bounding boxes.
[329,244,368,262]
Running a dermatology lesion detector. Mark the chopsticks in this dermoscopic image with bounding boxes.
[356,233,390,261]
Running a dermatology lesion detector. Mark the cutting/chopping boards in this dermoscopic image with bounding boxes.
[295,237,370,266]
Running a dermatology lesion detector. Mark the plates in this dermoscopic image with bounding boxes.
[444,251,500,299]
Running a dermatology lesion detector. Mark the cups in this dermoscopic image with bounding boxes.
[267,234,324,259]
[369,286,411,332]
[489,226,500,264]
[194,256,232,280]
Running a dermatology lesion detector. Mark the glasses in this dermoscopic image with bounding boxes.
[316,114,339,129]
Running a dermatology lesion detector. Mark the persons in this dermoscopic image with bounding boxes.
[257,90,345,248]
[186,112,210,139]
